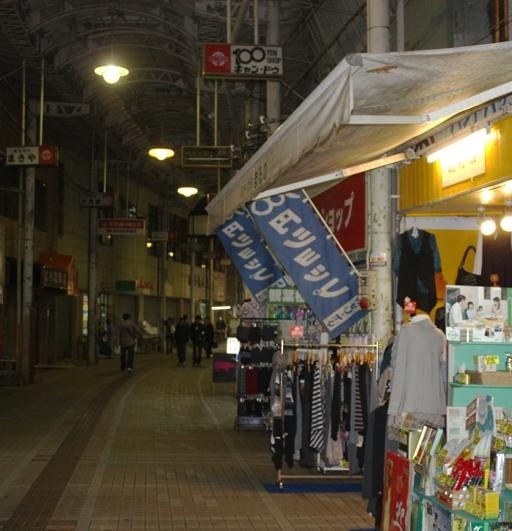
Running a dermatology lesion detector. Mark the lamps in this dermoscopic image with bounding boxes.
[87,3,131,86]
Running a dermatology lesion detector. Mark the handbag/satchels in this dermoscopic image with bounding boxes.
[456,266,480,285]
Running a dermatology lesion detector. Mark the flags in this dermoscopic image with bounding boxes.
[243,192,371,341]
[212,214,291,306]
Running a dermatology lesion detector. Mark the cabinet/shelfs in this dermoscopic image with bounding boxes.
[234,317,280,434]
[265,298,373,343]
[382,335,511,531]
[210,346,237,385]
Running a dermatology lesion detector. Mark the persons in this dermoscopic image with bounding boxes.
[95,303,240,375]
[446,287,503,327]
[387,312,448,430]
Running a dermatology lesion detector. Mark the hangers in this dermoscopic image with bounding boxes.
[287,344,378,378]
